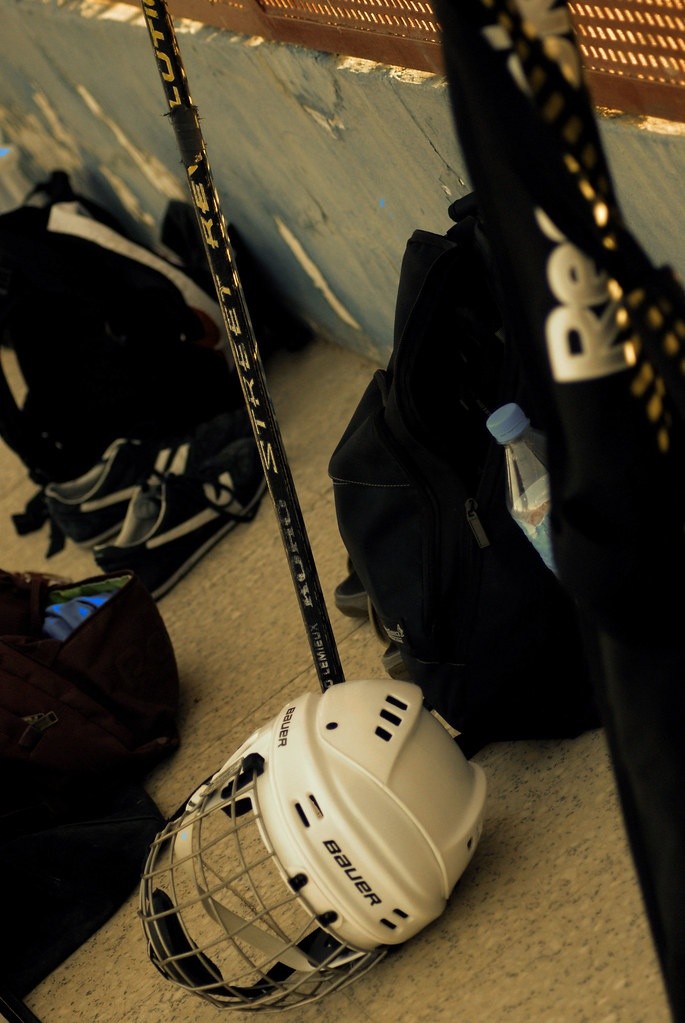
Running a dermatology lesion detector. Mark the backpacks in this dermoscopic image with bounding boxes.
[329,192,616,746]
[0,170,248,489]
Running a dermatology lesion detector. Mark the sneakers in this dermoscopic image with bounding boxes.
[47,440,179,552]
[93,439,269,602]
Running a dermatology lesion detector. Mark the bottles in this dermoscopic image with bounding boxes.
[487,403,557,574]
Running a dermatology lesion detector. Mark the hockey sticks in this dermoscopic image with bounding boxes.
[142,0,345,698]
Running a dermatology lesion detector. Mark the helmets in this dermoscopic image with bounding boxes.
[136,675,488,1013]
